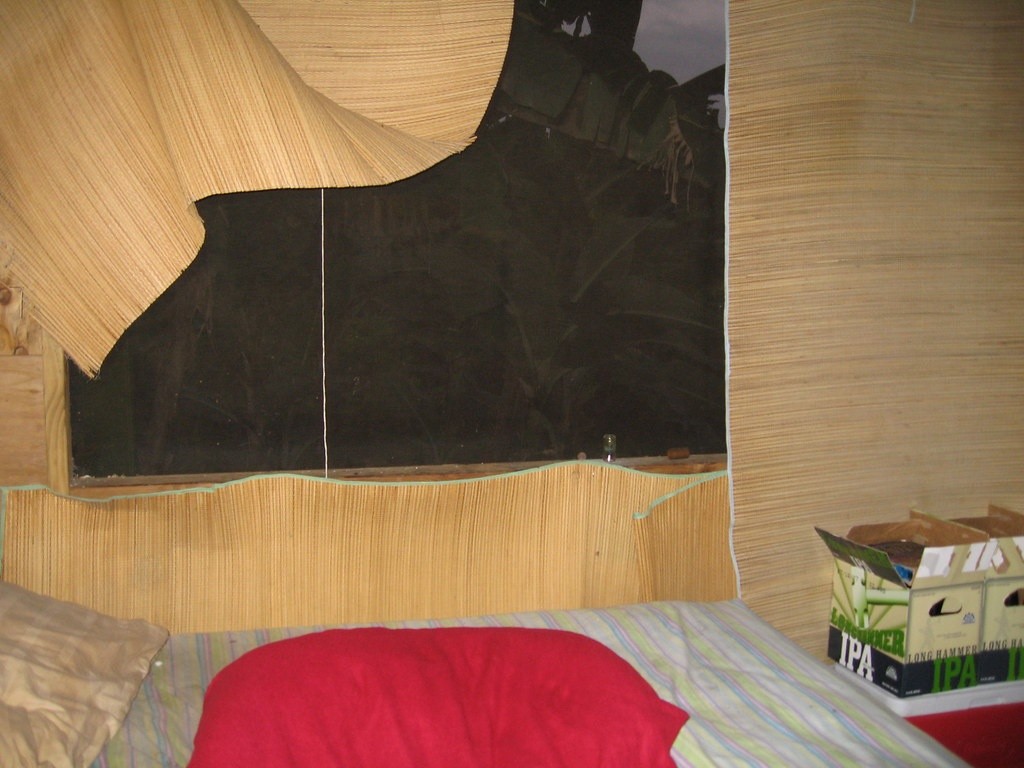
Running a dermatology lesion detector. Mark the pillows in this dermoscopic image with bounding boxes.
[0,577,168,768]
[185,628,693,768]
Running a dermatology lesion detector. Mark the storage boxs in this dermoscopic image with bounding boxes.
[812,503,1024,700]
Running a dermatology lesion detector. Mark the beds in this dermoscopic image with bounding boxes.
[87,597,978,768]
